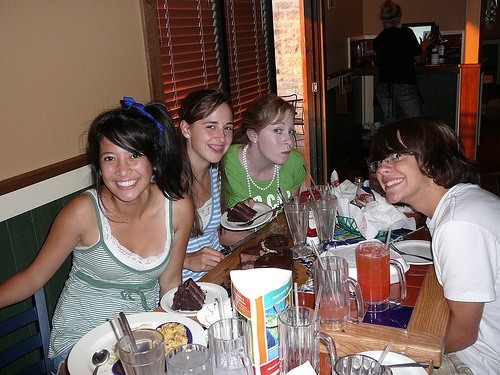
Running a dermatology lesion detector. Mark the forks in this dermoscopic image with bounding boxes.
[236,203,284,227]
[388,243,434,261]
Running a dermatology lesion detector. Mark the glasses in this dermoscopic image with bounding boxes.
[370,150,421,173]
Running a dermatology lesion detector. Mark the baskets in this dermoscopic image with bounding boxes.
[338,215,388,237]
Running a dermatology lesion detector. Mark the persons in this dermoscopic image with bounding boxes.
[372,0,435,125]
[0,96,195,373]
[176,90,270,299]
[363,117,500,375]
[218,92,307,232]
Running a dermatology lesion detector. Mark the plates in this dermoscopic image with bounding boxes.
[67,312,207,375]
[390,240,434,265]
[325,240,411,291]
[196,304,233,326]
[160,282,228,316]
[359,351,427,374]
[220,203,273,230]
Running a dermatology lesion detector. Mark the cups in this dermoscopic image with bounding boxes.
[334,353,393,375]
[282,185,338,256]
[166,344,210,375]
[208,318,254,375]
[277,306,337,375]
[313,256,364,331]
[355,240,407,312]
[116,328,165,375]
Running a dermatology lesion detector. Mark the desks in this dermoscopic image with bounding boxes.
[154,190,455,375]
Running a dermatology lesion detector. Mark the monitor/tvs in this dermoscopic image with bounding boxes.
[401,22,436,48]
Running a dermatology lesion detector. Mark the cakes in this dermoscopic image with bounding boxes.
[226,202,257,222]
[239,243,294,278]
[172,278,206,311]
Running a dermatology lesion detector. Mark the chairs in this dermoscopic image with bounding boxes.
[0,282,56,375]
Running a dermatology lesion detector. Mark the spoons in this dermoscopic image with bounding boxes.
[92,349,110,375]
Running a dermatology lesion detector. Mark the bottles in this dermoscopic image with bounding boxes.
[431,49,437,64]
[354,177,364,188]
[438,42,446,58]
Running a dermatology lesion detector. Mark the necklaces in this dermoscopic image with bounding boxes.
[242,143,276,190]
[243,144,279,232]
